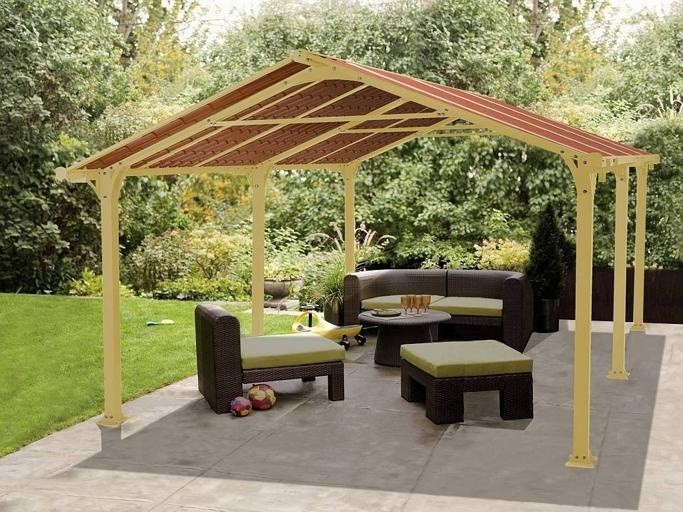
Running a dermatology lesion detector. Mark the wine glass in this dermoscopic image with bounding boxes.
[399,295,432,318]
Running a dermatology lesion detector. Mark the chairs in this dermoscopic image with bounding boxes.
[193,302,346,415]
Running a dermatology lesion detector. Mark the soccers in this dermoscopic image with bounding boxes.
[247,383,277,410]
[230,396,251,416]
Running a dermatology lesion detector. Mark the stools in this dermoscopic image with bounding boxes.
[399,339,533,425]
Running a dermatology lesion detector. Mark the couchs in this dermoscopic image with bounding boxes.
[341,268,534,354]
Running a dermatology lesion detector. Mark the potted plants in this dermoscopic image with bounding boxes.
[529,202,567,333]
[263,226,305,298]
